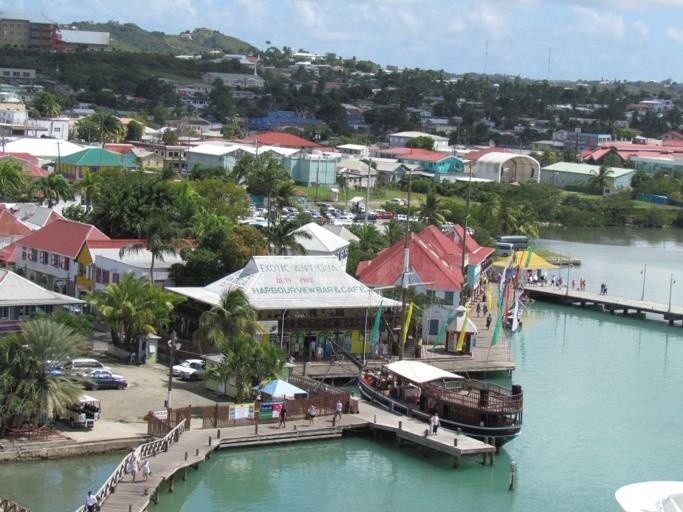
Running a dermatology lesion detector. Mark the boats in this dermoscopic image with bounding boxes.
[353,355,526,456]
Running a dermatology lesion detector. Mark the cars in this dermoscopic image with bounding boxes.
[232,190,474,240]
[21,349,209,431]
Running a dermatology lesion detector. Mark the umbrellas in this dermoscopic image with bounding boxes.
[260,378,308,397]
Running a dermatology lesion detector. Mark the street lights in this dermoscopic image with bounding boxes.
[553,251,677,313]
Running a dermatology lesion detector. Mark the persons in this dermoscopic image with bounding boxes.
[85,491,98,512]
[276,338,443,435]
[527,272,608,296]
[139,459,152,482]
[128,451,140,482]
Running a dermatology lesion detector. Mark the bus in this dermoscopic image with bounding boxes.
[495,235,530,250]
[493,242,514,258]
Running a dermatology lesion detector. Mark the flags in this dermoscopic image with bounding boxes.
[368,241,536,352]
